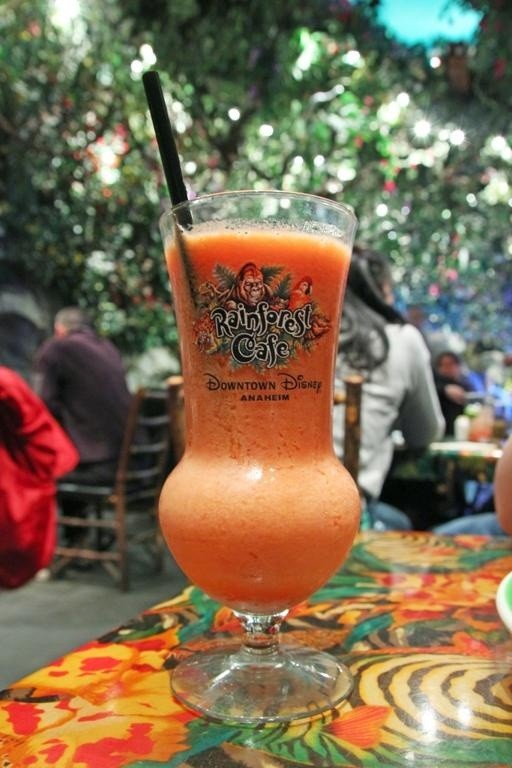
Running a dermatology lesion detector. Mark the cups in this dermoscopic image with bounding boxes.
[462,391,494,443]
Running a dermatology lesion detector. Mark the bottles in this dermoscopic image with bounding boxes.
[453,414,471,443]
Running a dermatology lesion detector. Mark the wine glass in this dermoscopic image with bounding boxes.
[156,187,363,728]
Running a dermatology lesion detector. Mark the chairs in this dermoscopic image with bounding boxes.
[332,372,366,488]
[46,375,189,595]
